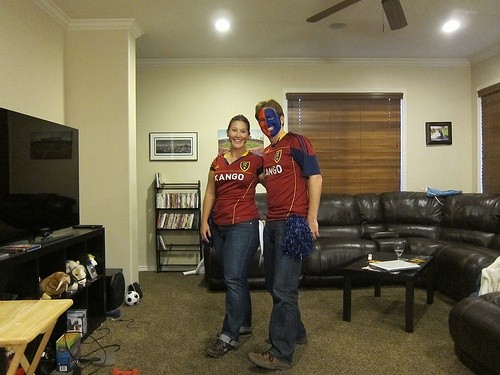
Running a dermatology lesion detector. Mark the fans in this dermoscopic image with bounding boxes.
[305,0,408,31]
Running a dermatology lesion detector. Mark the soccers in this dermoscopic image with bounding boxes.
[123,291,140,306]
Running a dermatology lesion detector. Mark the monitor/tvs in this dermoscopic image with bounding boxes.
[0,108,79,247]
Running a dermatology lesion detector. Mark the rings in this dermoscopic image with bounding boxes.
[312,235,315,237]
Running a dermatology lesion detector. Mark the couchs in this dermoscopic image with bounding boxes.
[204,191,500,375]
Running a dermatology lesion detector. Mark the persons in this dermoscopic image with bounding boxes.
[200,115,267,358]
[218,99,323,370]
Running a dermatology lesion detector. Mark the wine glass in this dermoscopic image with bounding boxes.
[394,239,406,259]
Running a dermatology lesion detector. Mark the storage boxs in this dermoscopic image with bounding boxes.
[56,331,80,372]
[68,309,87,338]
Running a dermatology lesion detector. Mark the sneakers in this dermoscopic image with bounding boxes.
[205,339,236,357]
[238,331,252,337]
[266,332,307,344]
[248,351,292,369]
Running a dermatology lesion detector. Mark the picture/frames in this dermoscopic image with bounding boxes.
[425,122,452,146]
[149,132,198,162]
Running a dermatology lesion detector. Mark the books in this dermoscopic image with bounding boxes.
[160,235,166,250]
[0,244,41,252]
[157,191,198,208]
[157,213,197,229]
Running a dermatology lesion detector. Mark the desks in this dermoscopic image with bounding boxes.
[0,299,73,375]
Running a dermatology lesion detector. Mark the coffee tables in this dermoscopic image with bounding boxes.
[343,252,434,333]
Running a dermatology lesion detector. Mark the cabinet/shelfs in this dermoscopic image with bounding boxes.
[0,226,107,375]
[154,180,202,273]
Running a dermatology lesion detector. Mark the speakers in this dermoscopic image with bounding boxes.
[104,268,125,312]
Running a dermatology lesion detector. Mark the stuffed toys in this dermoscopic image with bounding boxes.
[88,254,97,267]
[40,272,71,298]
[66,260,87,286]
